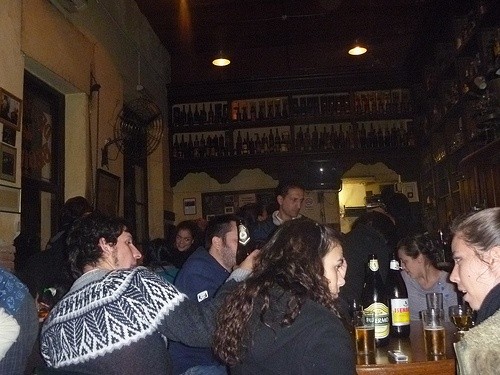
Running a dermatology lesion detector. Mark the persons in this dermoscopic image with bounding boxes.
[0,173,500,375]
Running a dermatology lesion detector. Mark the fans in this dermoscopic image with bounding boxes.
[99,96,164,172]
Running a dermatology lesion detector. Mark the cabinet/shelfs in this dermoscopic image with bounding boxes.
[411,0,500,176]
[167,72,421,189]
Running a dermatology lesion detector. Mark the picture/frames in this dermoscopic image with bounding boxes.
[1,125,17,146]
[237,190,257,209]
[0,184,21,214]
[94,168,120,218]
[224,205,234,215]
[0,87,24,130]
[181,197,197,215]
[401,180,420,204]
[205,214,217,222]
[0,142,18,182]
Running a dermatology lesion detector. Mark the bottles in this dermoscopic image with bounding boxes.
[360,253,391,347]
[171,89,415,157]
[384,246,411,337]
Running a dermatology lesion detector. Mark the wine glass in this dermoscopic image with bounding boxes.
[448,305,476,337]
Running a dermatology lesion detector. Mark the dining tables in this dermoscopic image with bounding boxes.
[345,317,460,375]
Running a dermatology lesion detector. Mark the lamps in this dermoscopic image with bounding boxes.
[346,37,369,56]
[210,49,232,67]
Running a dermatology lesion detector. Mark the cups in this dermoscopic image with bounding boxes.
[425,293,444,309]
[419,307,446,354]
[352,311,377,354]
[347,296,362,321]
